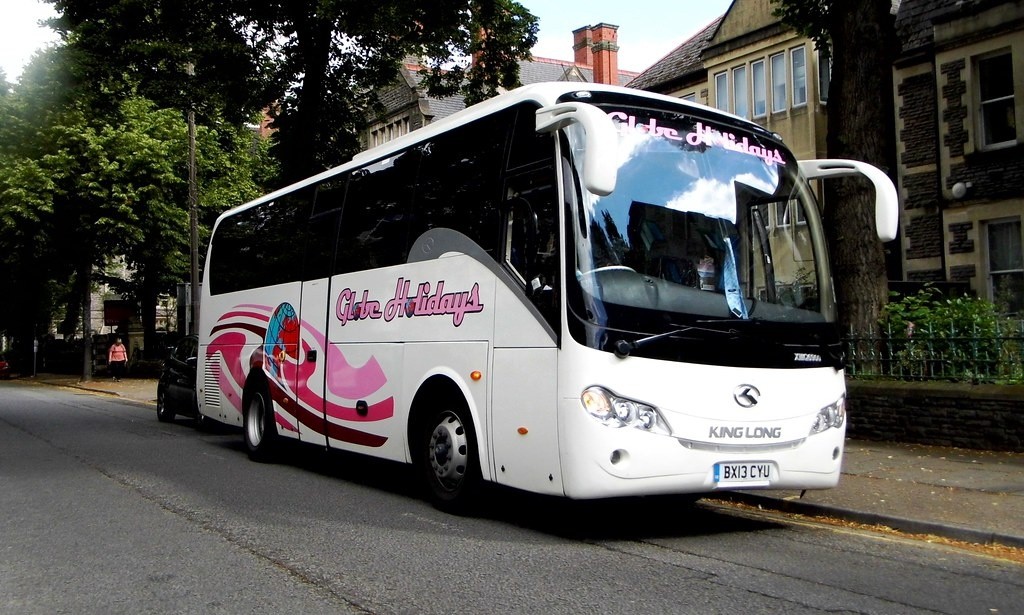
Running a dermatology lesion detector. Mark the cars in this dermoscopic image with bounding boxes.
[0,355,13,380]
[157,333,231,433]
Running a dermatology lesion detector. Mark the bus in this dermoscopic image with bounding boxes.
[195,81,897,517]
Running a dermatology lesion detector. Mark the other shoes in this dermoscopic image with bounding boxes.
[112,377,123,382]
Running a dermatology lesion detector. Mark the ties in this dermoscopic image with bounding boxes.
[724,239,748,319]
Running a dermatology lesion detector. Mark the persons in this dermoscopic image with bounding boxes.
[108,336,128,382]
